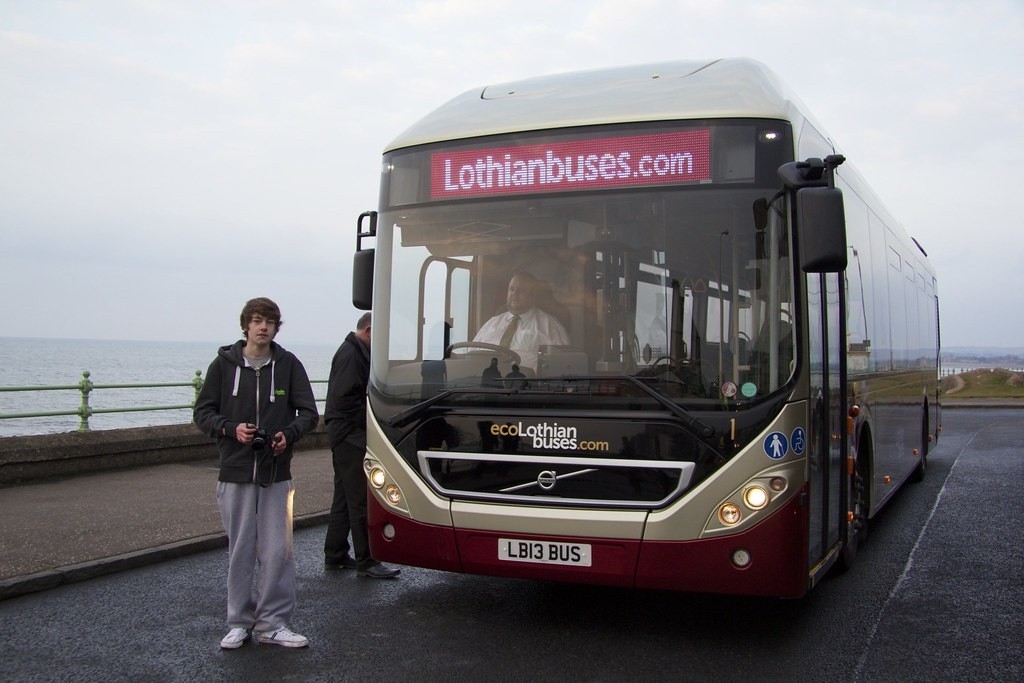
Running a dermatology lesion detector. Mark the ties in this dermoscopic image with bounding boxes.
[499,315,521,349]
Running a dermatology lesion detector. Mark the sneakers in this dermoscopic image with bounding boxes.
[220,627,249,647]
[257,626,309,647]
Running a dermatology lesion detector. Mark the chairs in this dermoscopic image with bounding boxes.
[752,309,792,394]
[728,331,751,378]
[494,279,572,335]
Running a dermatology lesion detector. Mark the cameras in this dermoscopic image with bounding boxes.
[247,426,278,451]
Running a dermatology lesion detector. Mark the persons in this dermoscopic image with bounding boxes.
[323,312,402,582]
[412,354,531,473]
[189,296,321,649]
[464,269,568,381]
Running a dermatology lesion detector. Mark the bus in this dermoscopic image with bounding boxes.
[350,65,945,602]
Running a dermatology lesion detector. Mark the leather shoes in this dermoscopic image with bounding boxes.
[324,558,357,569]
[358,563,401,579]
